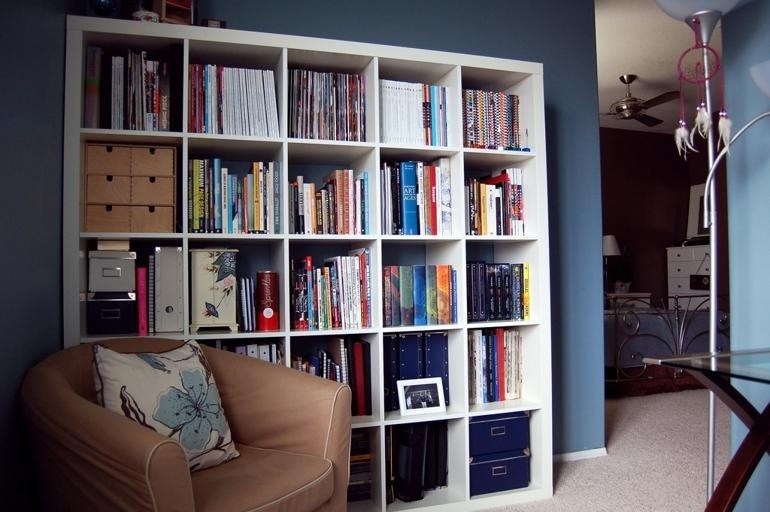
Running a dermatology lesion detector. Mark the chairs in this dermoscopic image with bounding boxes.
[19,336,353,512]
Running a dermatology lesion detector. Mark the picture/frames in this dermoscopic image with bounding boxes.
[396,377,447,417]
[686,183,710,239]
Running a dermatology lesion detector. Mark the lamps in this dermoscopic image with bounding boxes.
[657,0,770,505]
[602,234,622,293]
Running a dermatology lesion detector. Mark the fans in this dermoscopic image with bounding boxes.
[600,72,682,126]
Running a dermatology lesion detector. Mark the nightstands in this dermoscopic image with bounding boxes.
[606,292,651,309]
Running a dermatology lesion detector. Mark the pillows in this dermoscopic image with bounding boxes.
[91,340,239,476]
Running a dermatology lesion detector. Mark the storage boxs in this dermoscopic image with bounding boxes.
[469,453,531,498]
[469,411,530,456]
[84,142,177,233]
[88,248,137,293]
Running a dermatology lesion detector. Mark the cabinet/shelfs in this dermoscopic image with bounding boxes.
[666,245,710,310]
[63,15,554,512]
